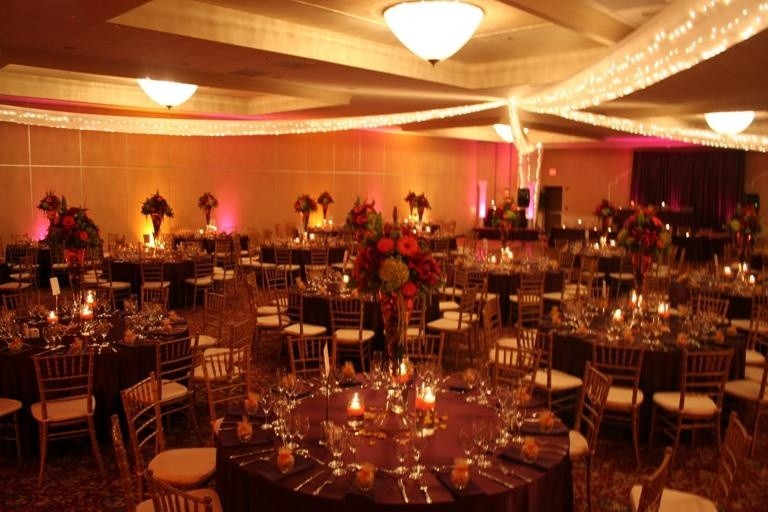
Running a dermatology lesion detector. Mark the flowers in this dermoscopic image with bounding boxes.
[594,199,672,286]
[490,198,521,244]
[294,188,459,374]
[140,187,176,237]
[35,191,104,304]
[195,190,218,224]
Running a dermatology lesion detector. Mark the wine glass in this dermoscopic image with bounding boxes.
[256,352,525,480]
[561,295,723,349]
[305,269,345,296]
[1,295,175,351]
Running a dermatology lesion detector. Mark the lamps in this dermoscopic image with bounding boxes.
[134,78,199,110]
[377,1,484,68]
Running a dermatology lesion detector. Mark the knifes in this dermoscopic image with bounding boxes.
[292,471,323,492]
[477,471,514,489]
[229,448,274,460]
[397,479,409,504]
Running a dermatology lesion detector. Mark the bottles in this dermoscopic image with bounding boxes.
[237,420,252,444]
[244,394,256,416]
[521,437,538,465]
[539,411,552,434]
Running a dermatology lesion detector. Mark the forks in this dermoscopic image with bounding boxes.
[311,473,336,496]
[418,478,433,505]
[497,464,533,484]
